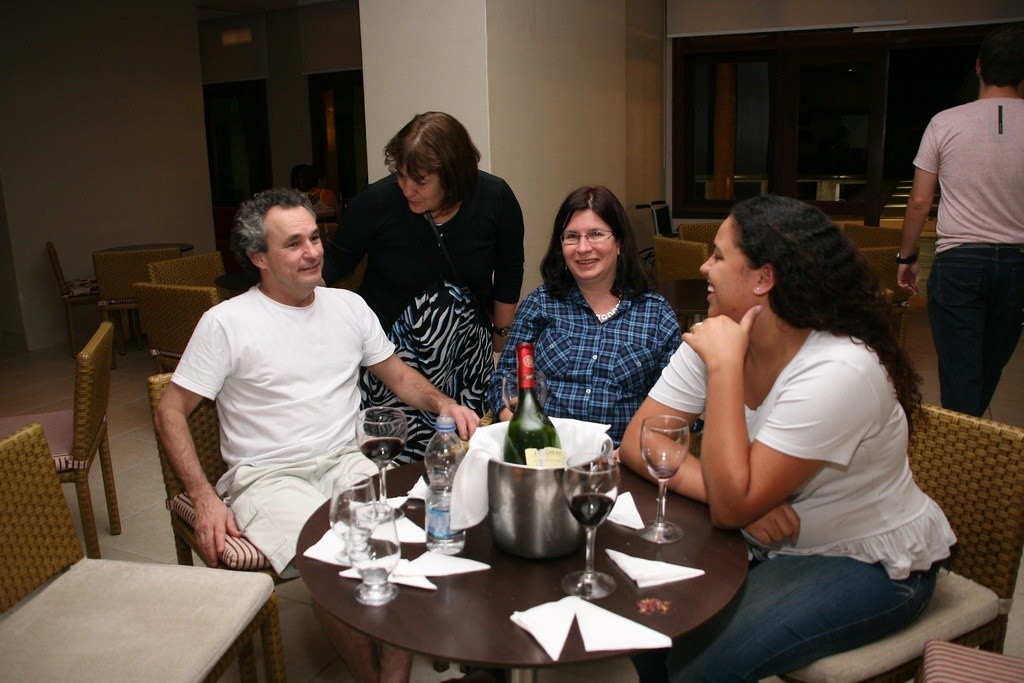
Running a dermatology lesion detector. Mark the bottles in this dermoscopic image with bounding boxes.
[502,342,564,471]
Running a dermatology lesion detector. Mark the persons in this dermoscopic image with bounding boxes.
[487,184,704,454]
[898,26,1024,416]
[619,194,957,683]
[322,111,524,465]
[290,163,352,215]
[154,187,481,683]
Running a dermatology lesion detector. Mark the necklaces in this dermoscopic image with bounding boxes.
[596,299,621,320]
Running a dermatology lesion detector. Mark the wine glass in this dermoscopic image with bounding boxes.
[328,473,377,563]
[424,416,466,556]
[638,416,684,543]
[349,504,400,606]
[561,453,617,600]
[355,408,408,523]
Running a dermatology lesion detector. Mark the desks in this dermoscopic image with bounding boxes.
[294,453,750,683]
[694,172,866,201]
[96,243,194,252]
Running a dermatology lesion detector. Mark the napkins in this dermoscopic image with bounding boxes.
[302,475,492,591]
[510,595,671,661]
[604,549,705,588]
[606,491,645,529]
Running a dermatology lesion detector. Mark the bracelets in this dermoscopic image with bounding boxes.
[897,252,919,263]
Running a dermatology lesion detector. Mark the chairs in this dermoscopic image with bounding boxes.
[147,372,450,683]
[316,221,369,293]
[776,397,1024,683]
[651,218,921,349]
[46,206,253,377]
[51,321,122,559]
[0,422,288,683]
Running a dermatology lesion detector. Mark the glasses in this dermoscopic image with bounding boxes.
[558,230,618,245]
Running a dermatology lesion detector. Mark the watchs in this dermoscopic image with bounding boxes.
[493,326,511,337]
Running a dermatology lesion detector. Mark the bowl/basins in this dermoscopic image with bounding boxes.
[473,423,613,558]
